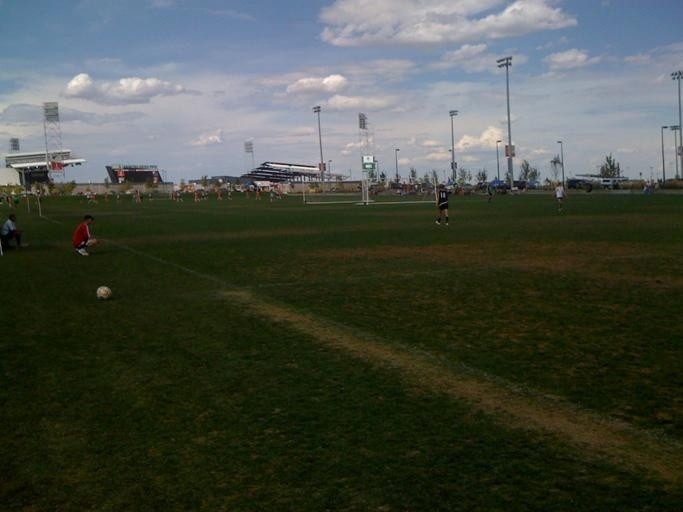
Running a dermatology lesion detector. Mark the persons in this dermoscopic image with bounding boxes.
[0,187,284,209]
[73,214,99,256]
[434,184,449,226]
[487,182,493,202]
[0,213,26,248]
[554,181,567,216]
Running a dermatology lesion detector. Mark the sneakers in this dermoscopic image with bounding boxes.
[74,246,88,255]
[435,220,448,225]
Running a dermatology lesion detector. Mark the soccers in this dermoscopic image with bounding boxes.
[96,286,111,300]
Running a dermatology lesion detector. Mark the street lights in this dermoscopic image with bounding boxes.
[556,141,566,191]
[395,148,400,184]
[358,112,371,176]
[661,70,683,184]
[244,141,255,169]
[448,109,459,181]
[312,106,325,182]
[496,56,514,191]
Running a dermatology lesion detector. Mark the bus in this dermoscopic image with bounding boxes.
[601,178,628,190]
